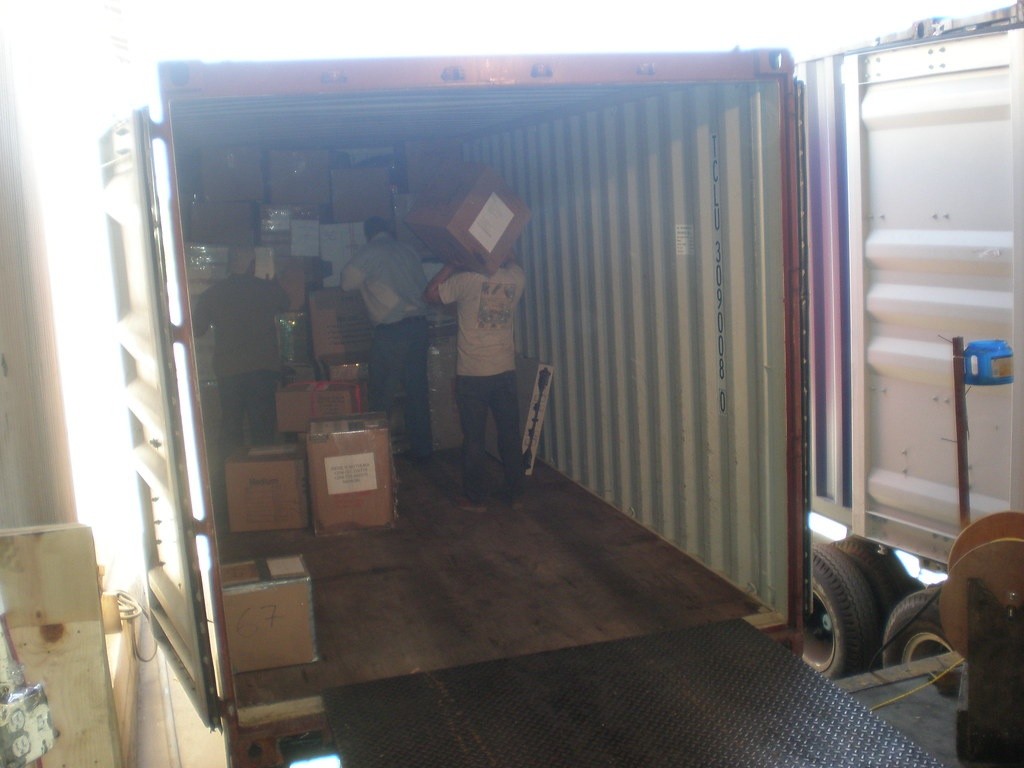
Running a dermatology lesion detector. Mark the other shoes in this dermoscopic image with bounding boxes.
[508,486,528,510]
[450,494,488,515]
[402,448,433,464]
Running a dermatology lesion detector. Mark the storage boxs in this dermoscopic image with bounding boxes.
[174,139,513,675]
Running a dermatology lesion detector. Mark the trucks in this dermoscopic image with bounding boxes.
[89,1,811,768]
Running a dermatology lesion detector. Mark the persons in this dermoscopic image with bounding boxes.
[192,245,289,485]
[341,215,433,465]
[421,247,528,515]
